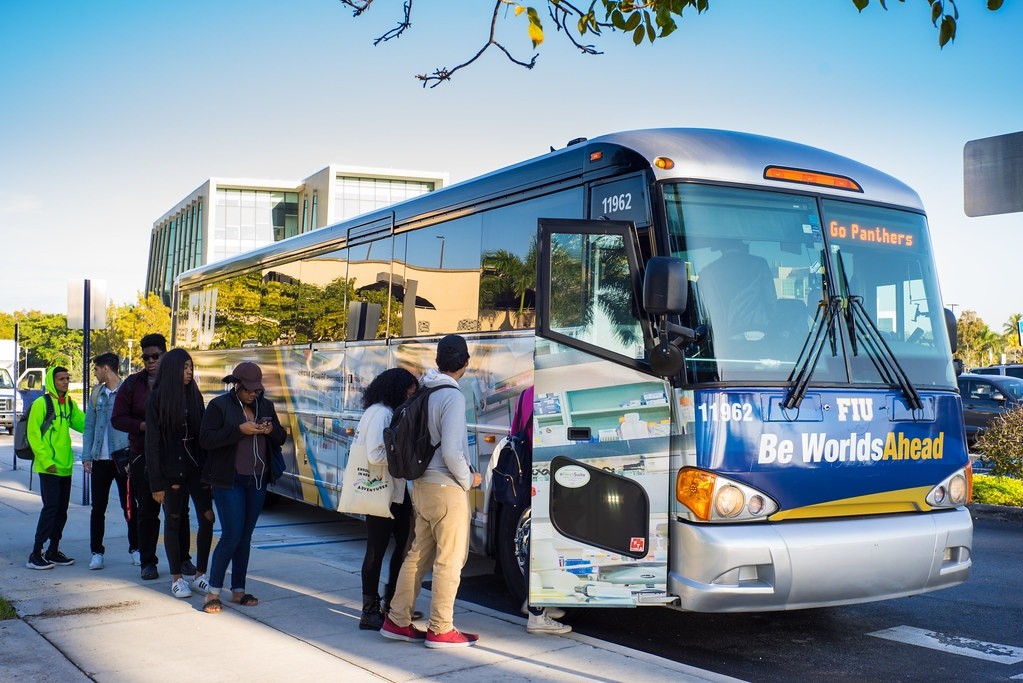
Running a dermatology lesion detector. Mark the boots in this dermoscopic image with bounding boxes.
[383,584,424,619]
[359,592,383,631]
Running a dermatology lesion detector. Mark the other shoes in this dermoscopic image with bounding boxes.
[140,564,160,579]
[181,558,197,574]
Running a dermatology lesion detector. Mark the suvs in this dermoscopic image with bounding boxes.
[957,363,1023,454]
[0,368,46,435]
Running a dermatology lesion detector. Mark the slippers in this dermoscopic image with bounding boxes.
[204,599,223,614]
[231,593,258,605]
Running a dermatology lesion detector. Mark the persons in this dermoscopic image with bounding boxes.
[143,349,216,598]
[380,332,482,648]
[695,238,782,362]
[508,349,572,634]
[336,367,424,631]
[110,333,197,580]
[201,361,285,614]
[26,365,86,569]
[799,285,831,339]
[81,353,142,569]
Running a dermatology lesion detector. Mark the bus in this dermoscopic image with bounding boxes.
[168,129,973,612]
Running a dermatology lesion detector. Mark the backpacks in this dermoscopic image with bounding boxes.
[487,390,534,507]
[383,385,460,480]
[14,394,72,460]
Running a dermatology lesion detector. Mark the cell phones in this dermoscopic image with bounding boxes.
[257,417,272,425]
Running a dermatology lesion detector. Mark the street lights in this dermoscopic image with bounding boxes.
[436,236,445,268]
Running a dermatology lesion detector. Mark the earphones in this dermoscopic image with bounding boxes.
[235,383,237,391]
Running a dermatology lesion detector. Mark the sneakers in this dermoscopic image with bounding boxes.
[526,608,572,634]
[378,612,427,642]
[172,578,192,597]
[131,550,141,565]
[26,553,55,569]
[44,550,75,565]
[90,552,104,569]
[425,626,480,647]
[522,599,565,618]
[192,574,210,593]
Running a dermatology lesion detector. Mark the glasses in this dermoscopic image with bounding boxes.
[141,351,164,361]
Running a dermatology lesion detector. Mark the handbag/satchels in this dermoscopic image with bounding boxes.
[112,448,144,477]
[335,407,394,520]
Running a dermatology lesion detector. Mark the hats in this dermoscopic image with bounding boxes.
[437,334,470,359]
[233,362,265,392]
